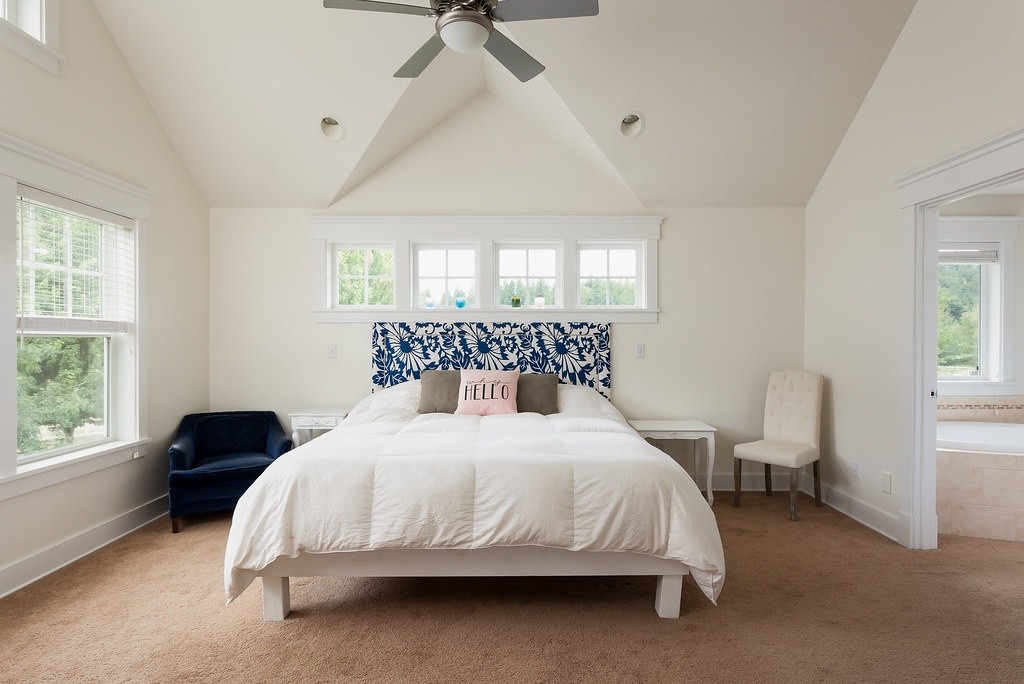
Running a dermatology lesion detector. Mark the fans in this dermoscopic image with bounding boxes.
[322,0,600,85]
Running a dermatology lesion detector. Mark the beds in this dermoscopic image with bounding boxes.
[224,322,727,622]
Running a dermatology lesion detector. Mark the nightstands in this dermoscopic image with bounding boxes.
[627,419,718,508]
[288,408,352,449]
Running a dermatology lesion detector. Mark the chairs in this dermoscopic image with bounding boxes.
[732,368,824,521]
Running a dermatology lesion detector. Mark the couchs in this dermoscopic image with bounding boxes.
[167,411,293,534]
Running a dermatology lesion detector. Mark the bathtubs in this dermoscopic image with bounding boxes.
[934,418,1023,543]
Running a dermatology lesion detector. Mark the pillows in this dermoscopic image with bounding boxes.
[418,370,560,417]
[453,365,520,416]
